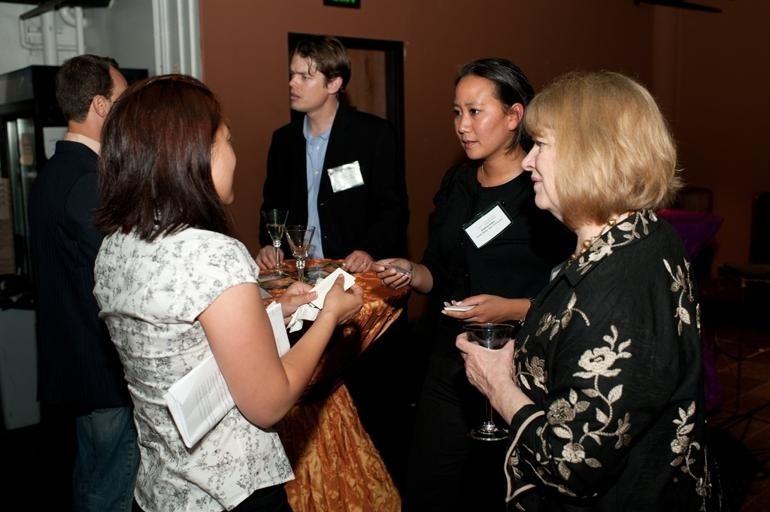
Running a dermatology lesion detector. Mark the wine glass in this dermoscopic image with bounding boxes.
[264,209,316,282]
[467,322,517,443]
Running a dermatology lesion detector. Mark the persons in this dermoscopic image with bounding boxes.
[93,70,366,511]
[455,67,723,512]
[23,51,141,511]
[370,55,578,512]
[255,33,416,512]
